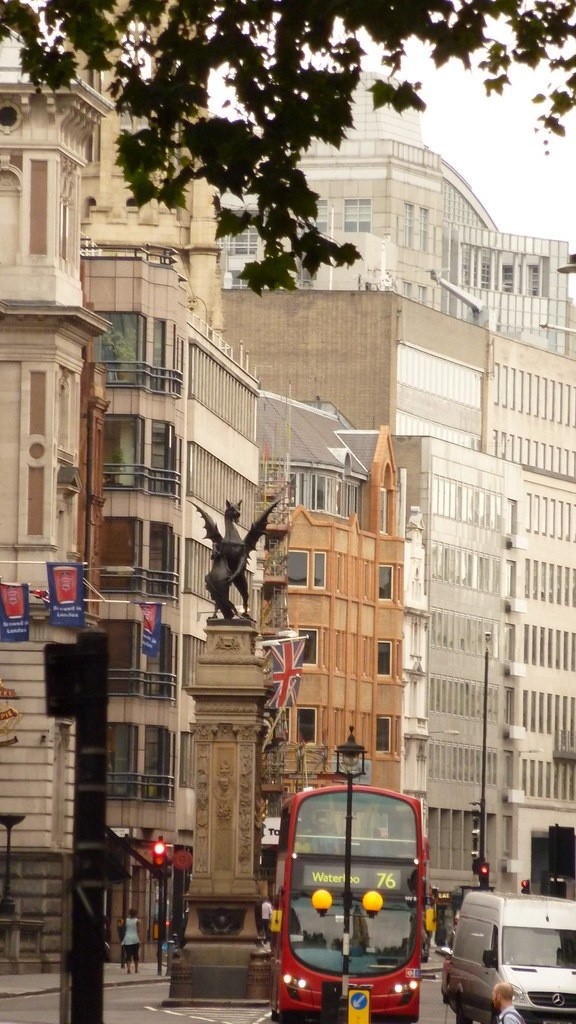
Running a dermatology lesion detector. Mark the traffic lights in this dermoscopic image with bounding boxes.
[479,863,489,889]
[521,880,530,894]
[153,840,167,866]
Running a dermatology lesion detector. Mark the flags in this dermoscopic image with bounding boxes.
[131,600,163,658]
[261,636,307,709]
[0,561,85,644]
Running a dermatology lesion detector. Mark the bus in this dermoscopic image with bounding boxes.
[270,783,430,1024]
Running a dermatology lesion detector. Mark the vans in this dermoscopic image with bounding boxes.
[440,888,576,1024]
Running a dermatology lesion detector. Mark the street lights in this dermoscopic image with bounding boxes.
[336,725,368,1024]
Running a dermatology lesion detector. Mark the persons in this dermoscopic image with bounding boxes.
[117,908,142,974]
[492,982,526,1024]
[435,921,448,947]
